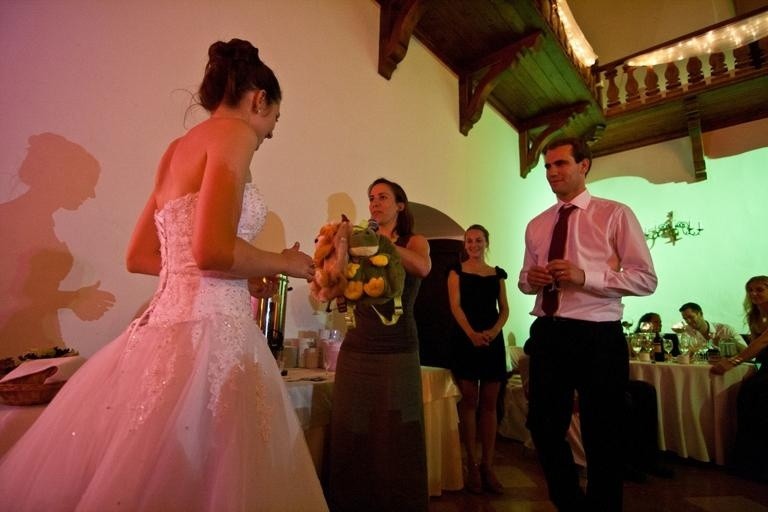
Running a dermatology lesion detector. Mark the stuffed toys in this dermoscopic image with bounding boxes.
[308,215,406,326]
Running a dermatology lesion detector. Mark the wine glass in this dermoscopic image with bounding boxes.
[622,318,656,363]
[663,337,710,365]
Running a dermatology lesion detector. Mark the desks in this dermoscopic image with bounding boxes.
[0,361,453,498]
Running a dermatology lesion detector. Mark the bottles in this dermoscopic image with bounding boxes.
[653,329,664,362]
[250,269,288,364]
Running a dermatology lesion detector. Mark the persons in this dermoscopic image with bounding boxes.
[0,40,330,511]
[516,350,676,484]
[633,312,672,360]
[324,179,434,512]
[515,133,658,511]
[709,275,767,486]
[678,302,749,358]
[445,223,510,496]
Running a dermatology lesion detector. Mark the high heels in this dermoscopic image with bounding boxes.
[467,463,505,495]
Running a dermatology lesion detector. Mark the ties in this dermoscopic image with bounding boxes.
[540,204,577,315]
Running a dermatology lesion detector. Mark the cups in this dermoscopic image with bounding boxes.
[283,336,319,369]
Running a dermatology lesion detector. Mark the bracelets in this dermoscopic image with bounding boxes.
[728,354,744,366]
[467,331,477,339]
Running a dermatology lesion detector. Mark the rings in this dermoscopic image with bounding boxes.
[559,270,564,275]
